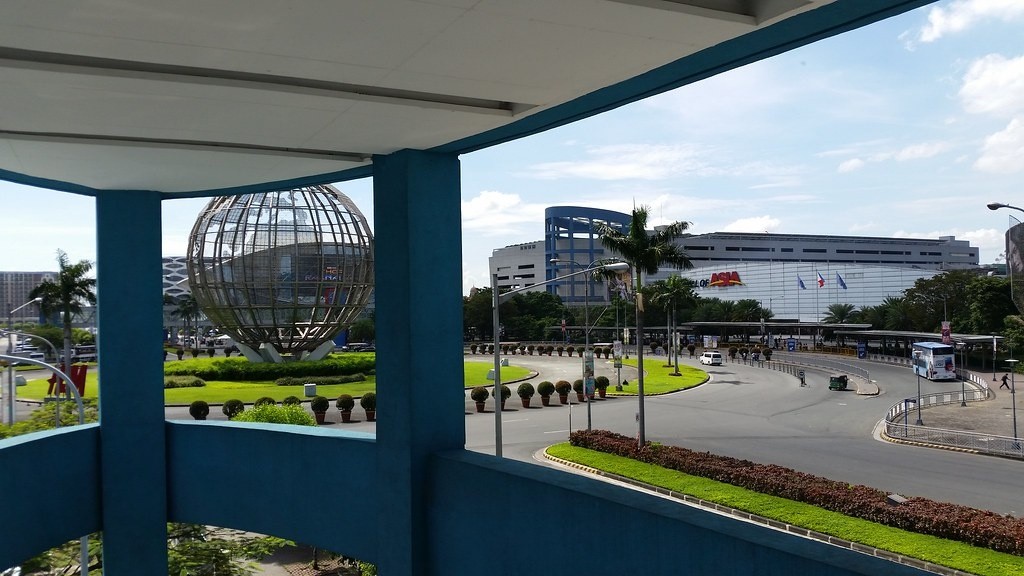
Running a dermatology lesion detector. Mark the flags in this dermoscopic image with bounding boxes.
[797,276,807,290]
[818,273,825,287]
[836,273,847,290]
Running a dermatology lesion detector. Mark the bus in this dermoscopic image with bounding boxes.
[911,342,956,381]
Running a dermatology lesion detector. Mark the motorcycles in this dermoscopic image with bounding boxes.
[829,374,849,391]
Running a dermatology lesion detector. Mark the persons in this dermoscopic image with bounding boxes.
[1000,373,1011,390]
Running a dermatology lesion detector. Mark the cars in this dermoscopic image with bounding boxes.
[184,337,222,347]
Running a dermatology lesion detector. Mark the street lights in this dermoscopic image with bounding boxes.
[1004,359,1022,450]
[491,263,630,455]
[914,349,924,426]
[957,342,968,407]
[549,258,620,432]
[6,298,44,428]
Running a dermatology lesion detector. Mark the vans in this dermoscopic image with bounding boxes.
[700,352,723,366]
